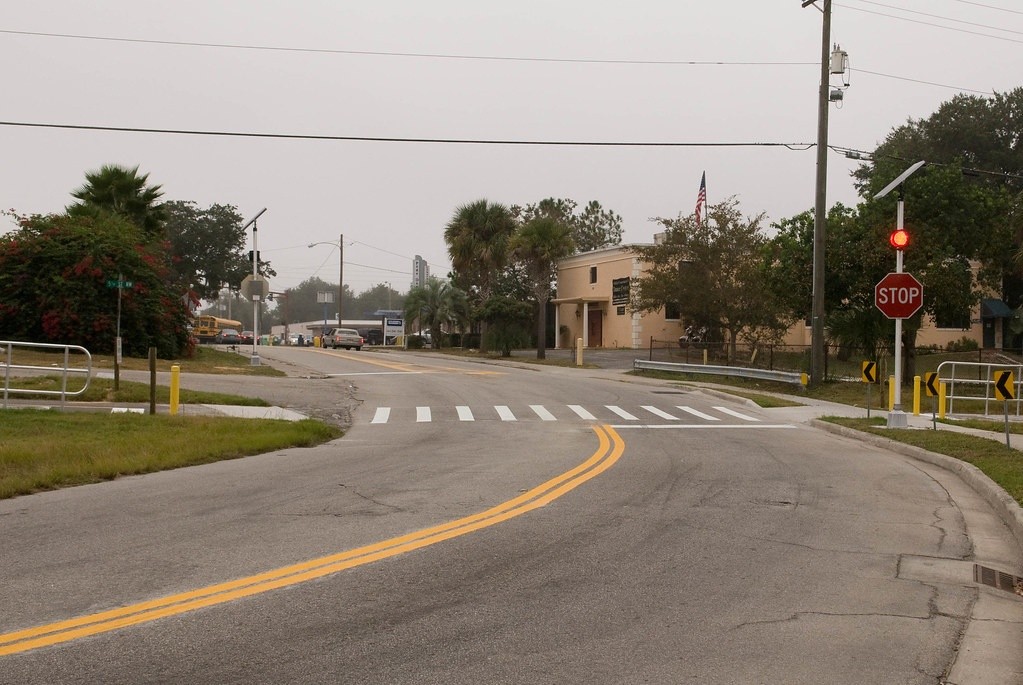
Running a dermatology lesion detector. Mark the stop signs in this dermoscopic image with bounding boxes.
[875,273,923,319]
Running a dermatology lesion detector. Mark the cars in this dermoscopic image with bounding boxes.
[321,328,364,351]
[411,328,431,344]
[240,331,260,345]
[280,333,314,347]
[214,329,241,344]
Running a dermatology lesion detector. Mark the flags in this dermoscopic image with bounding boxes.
[694,172,705,223]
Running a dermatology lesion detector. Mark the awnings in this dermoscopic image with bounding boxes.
[980,299,1012,318]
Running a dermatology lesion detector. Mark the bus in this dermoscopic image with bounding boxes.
[191,316,242,344]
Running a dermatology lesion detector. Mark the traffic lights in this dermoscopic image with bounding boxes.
[890,230,911,249]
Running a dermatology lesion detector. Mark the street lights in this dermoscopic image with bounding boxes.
[874,160,926,427]
[241,208,268,366]
[309,234,343,328]
[385,281,392,310]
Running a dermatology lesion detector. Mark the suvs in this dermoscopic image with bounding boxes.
[367,330,390,345]
